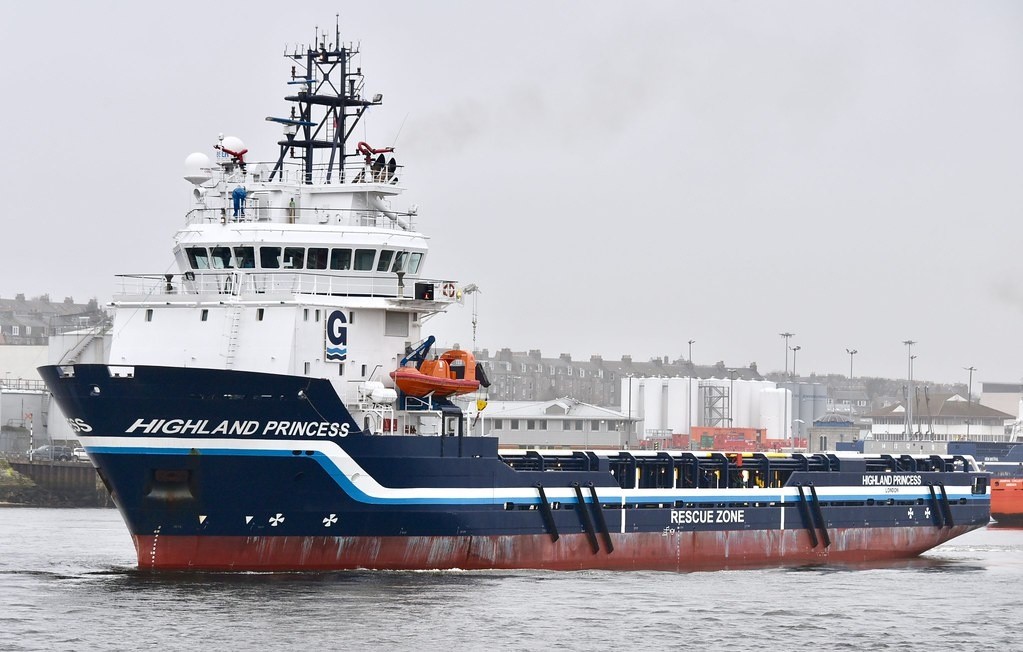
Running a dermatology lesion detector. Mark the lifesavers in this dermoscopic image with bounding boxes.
[443,284,455,297]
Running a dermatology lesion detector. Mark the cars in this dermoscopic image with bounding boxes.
[71,447,91,461]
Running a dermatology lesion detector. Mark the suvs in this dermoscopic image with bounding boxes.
[26,445,72,462]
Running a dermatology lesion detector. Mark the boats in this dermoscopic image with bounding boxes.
[34,14,993,574]
[947,439,1023,527]
[389,349,481,398]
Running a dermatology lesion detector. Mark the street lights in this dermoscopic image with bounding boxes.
[964,366,978,440]
[904,339,919,432]
[626,373,634,450]
[789,345,801,453]
[846,348,858,419]
[688,339,696,451]
[778,332,796,441]
[728,368,736,428]
